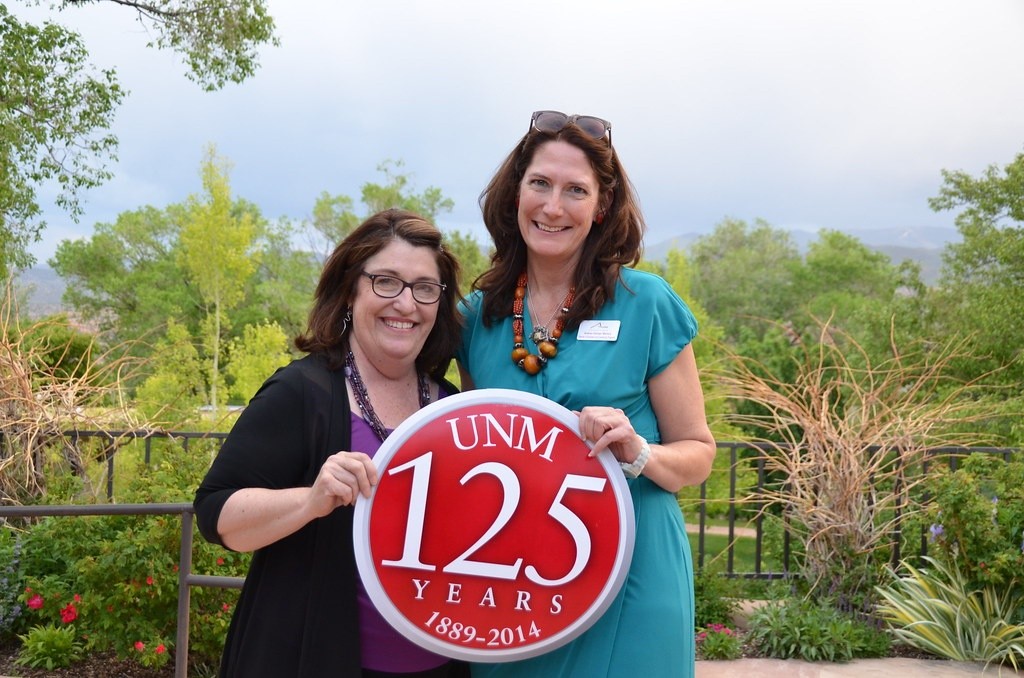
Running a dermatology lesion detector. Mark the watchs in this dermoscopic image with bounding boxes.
[619,434,650,480]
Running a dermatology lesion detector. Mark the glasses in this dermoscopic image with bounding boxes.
[529,111,611,149]
[360,270,447,305]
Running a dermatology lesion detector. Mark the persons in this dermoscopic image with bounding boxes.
[454,110,717,678]
[192,209,473,678]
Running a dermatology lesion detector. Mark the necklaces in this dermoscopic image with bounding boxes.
[511,271,577,375]
[527,281,570,344]
[345,346,432,443]
